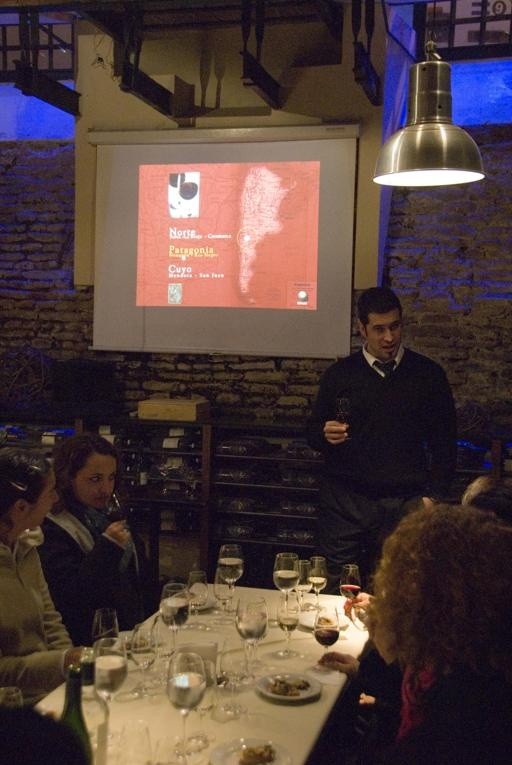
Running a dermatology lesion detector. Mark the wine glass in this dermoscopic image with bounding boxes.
[91,636,126,745]
[314,605,339,672]
[219,637,247,714]
[272,552,299,616]
[166,651,208,754]
[188,570,208,629]
[236,596,268,684]
[293,556,327,611]
[159,583,190,657]
[340,563,362,600]
[91,607,119,649]
[103,489,130,529]
[131,623,158,699]
[218,543,244,594]
[278,592,299,658]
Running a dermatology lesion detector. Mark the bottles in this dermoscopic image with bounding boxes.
[59,646,107,764]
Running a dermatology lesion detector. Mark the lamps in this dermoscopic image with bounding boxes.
[370,0,486,189]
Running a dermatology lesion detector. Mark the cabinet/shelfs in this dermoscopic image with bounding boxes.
[73,412,323,581]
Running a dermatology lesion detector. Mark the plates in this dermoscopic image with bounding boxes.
[257,671,321,702]
[208,737,294,764]
[299,609,349,630]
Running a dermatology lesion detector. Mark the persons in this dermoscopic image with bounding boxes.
[37,432,161,647]
[0,708,93,765]
[0,446,93,707]
[363,496,511,765]
[317,474,512,712]
[304,287,458,596]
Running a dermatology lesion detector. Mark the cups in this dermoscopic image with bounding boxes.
[334,398,354,440]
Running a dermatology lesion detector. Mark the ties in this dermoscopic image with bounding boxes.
[374,359,401,393]
